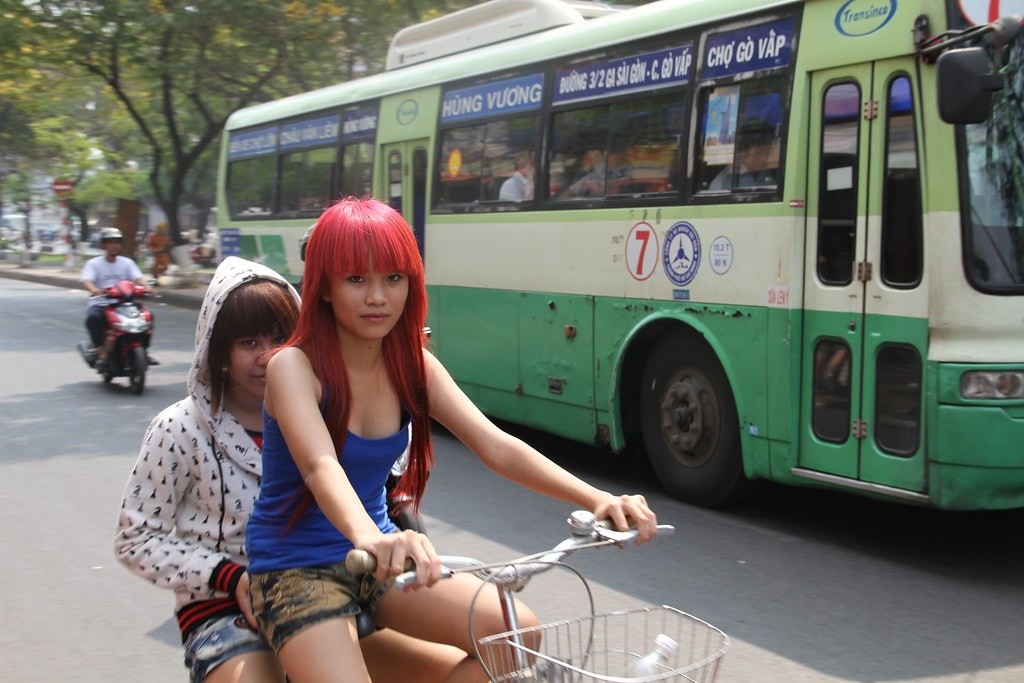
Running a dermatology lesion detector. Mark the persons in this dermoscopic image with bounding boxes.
[708,120,772,191]
[115,256,467,683]
[81,227,159,369]
[246,200,656,683]
[500,142,535,201]
[146,223,217,287]
[562,141,634,198]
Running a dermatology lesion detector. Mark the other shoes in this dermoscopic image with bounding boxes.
[96,349,107,364]
[147,357,160,365]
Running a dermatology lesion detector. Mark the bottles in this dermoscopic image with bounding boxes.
[625,634,679,683]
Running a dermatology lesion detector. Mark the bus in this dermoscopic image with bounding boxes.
[214,2,1024,515]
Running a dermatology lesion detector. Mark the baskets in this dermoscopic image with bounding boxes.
[464,608,733,683]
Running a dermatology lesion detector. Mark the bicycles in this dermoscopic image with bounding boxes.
[344,509,732,683]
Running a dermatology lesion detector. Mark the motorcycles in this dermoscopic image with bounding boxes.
[74,280,162,395]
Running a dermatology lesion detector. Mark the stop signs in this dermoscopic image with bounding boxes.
[52,179,76,196]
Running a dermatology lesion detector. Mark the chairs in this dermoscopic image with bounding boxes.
[480,176,511,201]
[443,177,479,202]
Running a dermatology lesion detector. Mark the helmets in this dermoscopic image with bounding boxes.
[99,227,122,241]
